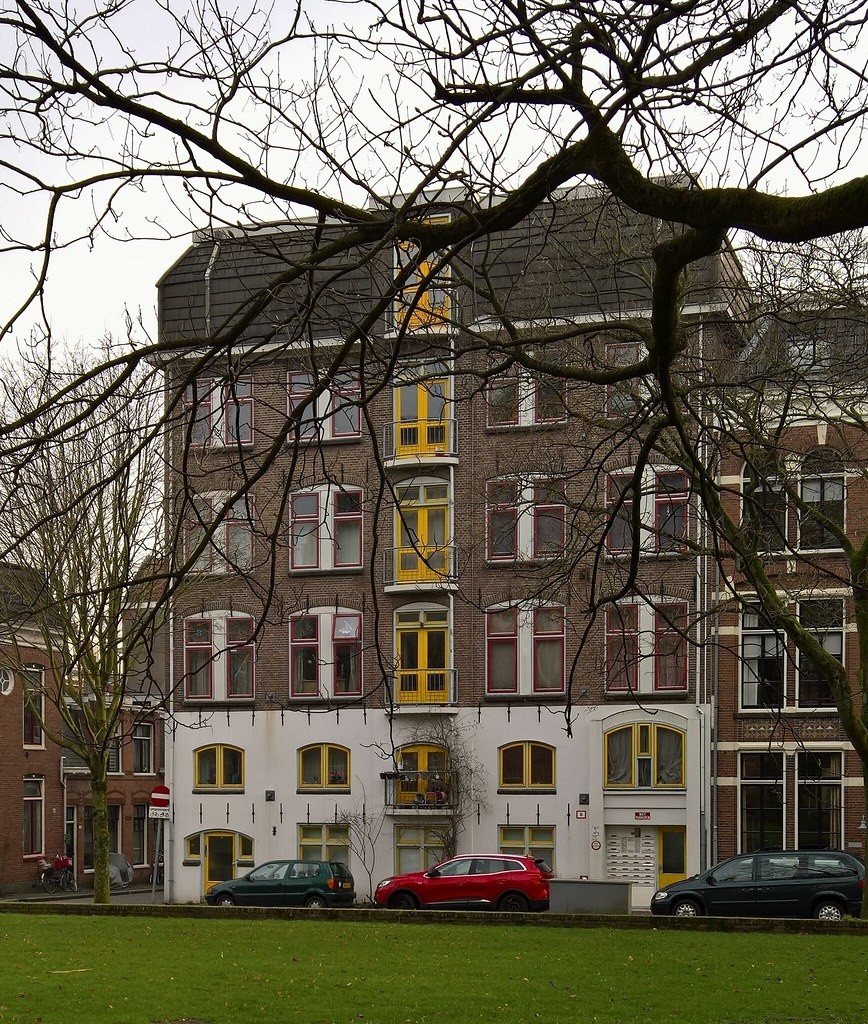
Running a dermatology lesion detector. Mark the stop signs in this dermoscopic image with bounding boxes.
[151,784,170,807]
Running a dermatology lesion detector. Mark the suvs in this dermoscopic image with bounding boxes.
[374,853,554,912]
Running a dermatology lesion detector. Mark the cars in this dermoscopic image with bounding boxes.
[649,850,866,921]
[205,859,357,909]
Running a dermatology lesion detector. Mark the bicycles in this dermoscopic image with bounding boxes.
[40,853,78,894]
